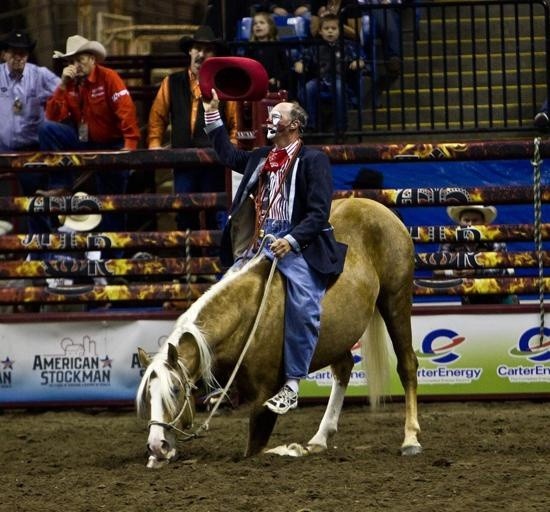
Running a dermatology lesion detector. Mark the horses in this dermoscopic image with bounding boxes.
[135,196,423,469]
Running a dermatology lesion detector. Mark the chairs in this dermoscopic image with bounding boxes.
[232,0,422,138]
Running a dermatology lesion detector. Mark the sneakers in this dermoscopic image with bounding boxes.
[262,387,298,415]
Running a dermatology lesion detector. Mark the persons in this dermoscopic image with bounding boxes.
[533,99,547,129]
[148,26,228,283]
[432,203,519,306]
[36,34,145,285]
[242,0,404,143]
[202,87,349,416]
[344,169,404,225]
[0,29,66,284]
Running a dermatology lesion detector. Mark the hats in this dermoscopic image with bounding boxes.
[200,56,268,101]
[447,205,497,224]
[344,168,386,192]
[179,25,222,53]
[53,34,106,63]
[0,31,37,53]
[65,192,102,232]
